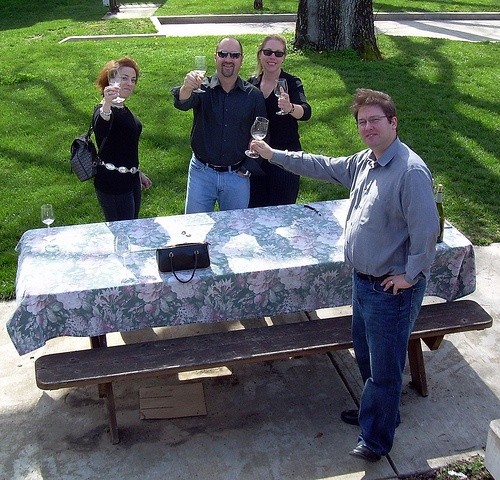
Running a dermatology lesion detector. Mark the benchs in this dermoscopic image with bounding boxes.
[35,300,494,444]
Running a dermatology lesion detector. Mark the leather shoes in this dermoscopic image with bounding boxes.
[353,443,381,463]
[342,411,360,424]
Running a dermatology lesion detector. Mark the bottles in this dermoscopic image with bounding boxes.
[435,183,444,243]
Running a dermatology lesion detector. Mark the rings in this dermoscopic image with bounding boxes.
[391,281,393,284]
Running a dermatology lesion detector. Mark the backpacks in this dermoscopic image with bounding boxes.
[71,107,114,182]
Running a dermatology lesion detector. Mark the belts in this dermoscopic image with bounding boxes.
[356,270,388,282]
[194,153,243,172]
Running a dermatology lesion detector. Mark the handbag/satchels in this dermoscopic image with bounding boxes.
[156,242,211,284]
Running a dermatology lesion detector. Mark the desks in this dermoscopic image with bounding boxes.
[5,199,477,398]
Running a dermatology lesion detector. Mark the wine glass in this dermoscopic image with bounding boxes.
[40,204,55,236]
[114,234,130,275]
[245,117,269,159]
[108,67,125,103]
[274,78,288,115]
[193,56,206,93]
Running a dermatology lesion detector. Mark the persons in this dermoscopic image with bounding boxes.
[251,88,441,463]
[93,57,152,222]
[171,35,311,214]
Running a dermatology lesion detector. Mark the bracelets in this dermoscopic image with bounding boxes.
[100,107,112,116]
[288,103,294,114]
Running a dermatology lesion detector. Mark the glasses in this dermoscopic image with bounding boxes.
[216,51,241,59]
[262,49,285,57]
[355,116,386,125]
